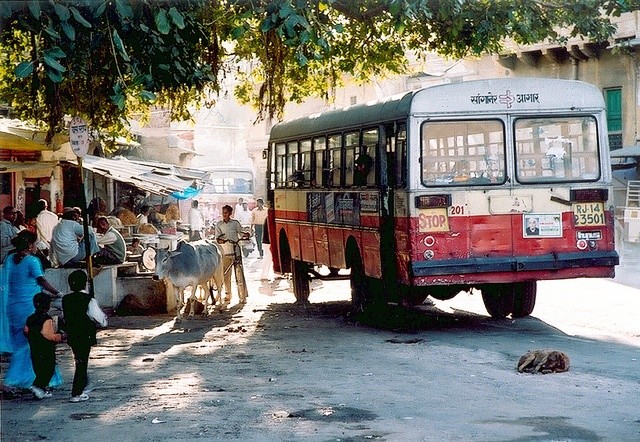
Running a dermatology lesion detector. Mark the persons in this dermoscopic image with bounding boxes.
[94,198,126,267]
[234,198,243,219]
[62,269,108,403]
[526,218,540,235]
[214,205,251,304]
[353,154,375,185]
[0,229,63,394]
[252,199,270,259]
[136,205,148,225]
[127,237,144,254]
[1,199,58,260]
[50,207,100,268]
[240,201,250,225]
[188,201,219,239]
[23,293,66,399]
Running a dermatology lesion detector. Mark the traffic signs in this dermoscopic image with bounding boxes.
[69,116,90,157]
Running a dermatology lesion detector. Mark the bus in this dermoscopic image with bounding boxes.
[263,76,619,318]
[146,166,257,224]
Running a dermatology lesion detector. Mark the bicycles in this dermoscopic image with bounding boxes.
[205,232,251,305]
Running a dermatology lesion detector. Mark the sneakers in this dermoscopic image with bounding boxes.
[69,393,89,403]
[43,390,54,398]
[27,385,45,399]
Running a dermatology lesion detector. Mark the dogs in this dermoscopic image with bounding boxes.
[517,350,571,374]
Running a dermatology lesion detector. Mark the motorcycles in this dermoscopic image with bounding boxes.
[239,219,255,257]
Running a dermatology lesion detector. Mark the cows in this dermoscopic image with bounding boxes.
[147,238,224,323]
